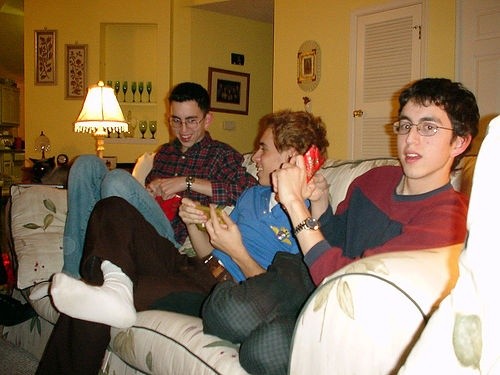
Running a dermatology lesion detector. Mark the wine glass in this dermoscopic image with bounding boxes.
[149,120,157,139]
[122,80,127,103]
[139,120,147,139]
[131,81,136,102]
[138,80,143,102]
[146,81,152,102]
[115,81,120,99]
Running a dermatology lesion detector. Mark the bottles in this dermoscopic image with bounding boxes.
[122,110,134,138]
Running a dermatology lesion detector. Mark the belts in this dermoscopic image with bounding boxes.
[206,255,233,284]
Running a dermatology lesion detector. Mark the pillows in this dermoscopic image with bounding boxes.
[9,184,68,289]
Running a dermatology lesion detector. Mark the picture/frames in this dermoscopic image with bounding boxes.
[34,29,57,86]
[208,66,250,115]
[63,44,88,100]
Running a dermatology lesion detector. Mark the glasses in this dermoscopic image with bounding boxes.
[171,116,204,129]
[393,122,455,136]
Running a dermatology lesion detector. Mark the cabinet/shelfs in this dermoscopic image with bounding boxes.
[105,100,159,143]
[0,84,20,126]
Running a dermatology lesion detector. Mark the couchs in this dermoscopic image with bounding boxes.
[3,151,478,375]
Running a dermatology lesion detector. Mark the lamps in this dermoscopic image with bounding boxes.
[34,131,51,160]
[73,80,128,159]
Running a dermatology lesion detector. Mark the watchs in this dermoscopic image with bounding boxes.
[185,176,195,192]
[294,218,319,234]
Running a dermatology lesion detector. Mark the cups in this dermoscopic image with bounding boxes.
[107,80,112,88]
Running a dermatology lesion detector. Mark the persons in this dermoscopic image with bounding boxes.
[202,79,480,375]
[36,111,329,375]
[61,82,259,280]
